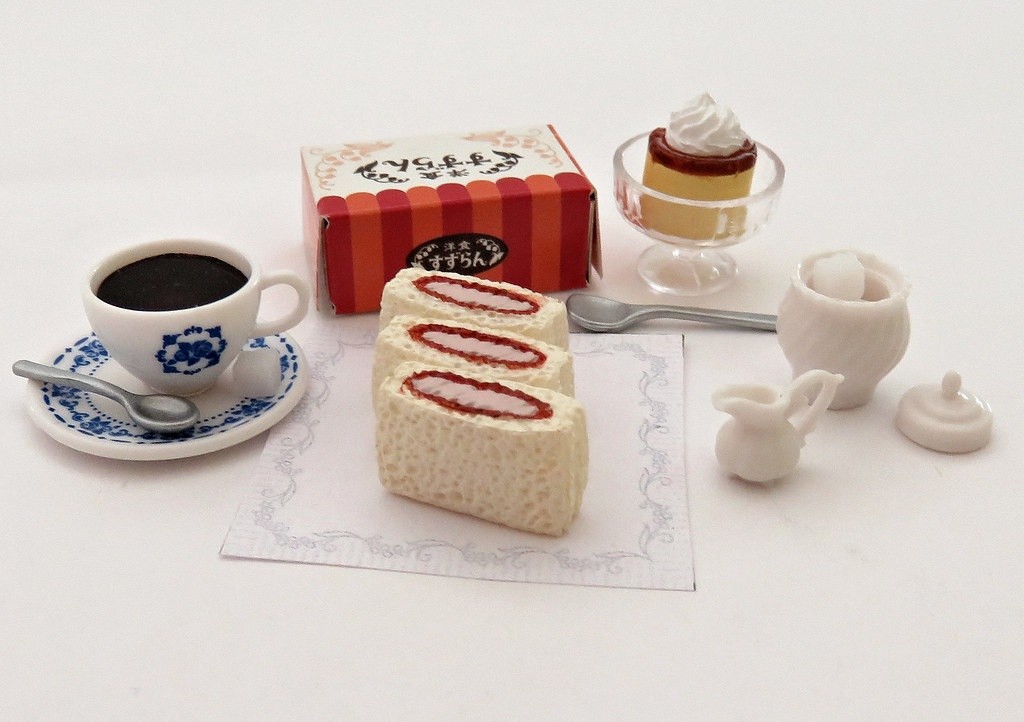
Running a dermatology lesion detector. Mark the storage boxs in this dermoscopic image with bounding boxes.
[301,123,603,315]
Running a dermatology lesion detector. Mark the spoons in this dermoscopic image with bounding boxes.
[12,359,197,434]
[566,292,777,335]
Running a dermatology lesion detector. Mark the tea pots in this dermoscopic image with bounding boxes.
[710,372,839,480]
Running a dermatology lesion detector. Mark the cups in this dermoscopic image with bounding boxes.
[780,252,909,408]
[85,237,308,396]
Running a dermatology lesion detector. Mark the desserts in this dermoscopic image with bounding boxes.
[637,90,758,242]
[371,266,592,536]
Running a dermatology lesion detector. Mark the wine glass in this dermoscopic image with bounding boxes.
[618,132,786,297]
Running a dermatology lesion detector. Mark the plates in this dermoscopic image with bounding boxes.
[26,328,307,460]
